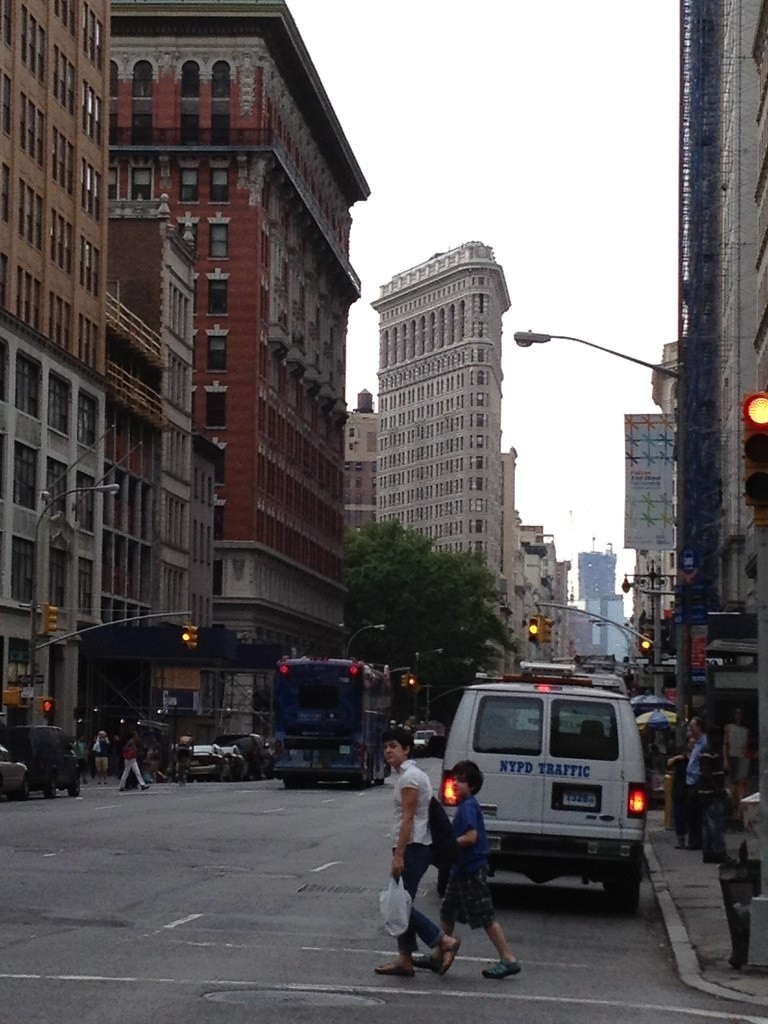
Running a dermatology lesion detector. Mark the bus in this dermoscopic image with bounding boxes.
[272,655,392,790]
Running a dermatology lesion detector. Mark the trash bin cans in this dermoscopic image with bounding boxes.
[718,858,761,968]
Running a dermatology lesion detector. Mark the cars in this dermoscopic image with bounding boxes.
[0,744,33,800]
[185,733,275,781]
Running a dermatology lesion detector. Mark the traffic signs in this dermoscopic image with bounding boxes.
[18,675,44,684]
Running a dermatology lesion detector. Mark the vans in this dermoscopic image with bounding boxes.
[413,730,436,746]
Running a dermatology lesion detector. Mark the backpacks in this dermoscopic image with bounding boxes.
[429,796,460,869]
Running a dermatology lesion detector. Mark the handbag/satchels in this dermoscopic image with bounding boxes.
[380,873,412,935]
[124,748,137,760]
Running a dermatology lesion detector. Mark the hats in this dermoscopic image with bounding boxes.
[381,726,413,745]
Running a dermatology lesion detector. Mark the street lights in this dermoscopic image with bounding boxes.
[29,483,120,727]
[513,330,703,832]
[414,648,444,724]
[182,625,192,644]
[622,558,678,812]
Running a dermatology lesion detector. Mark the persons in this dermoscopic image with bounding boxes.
[72,730,150,791]
[667,706,752,849]
[413,760,521,976]
[375,728,460,976]
[177,732,193,774]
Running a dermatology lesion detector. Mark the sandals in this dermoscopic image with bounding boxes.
[412,952,442,972]
[482,958,522,979]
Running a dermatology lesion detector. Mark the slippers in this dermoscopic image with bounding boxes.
[375,961,416,977]
[440,937,462,974]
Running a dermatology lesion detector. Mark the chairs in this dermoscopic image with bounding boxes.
[582,720,603,735]
[491,713,512,730]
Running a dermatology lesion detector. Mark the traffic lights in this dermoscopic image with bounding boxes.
[39,602,58,635]
[188,625,199,649]
[743,391,768,510]
[528,616,540,647]
[409,673,415,687]
[400,674,408,687]
[540,616,555,643]
[41,697,53,713]
[638,631,654,653]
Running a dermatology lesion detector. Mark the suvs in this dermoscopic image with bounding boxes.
[437,662,649,907]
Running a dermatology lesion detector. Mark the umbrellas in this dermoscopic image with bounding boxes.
[631,693,679,732]
